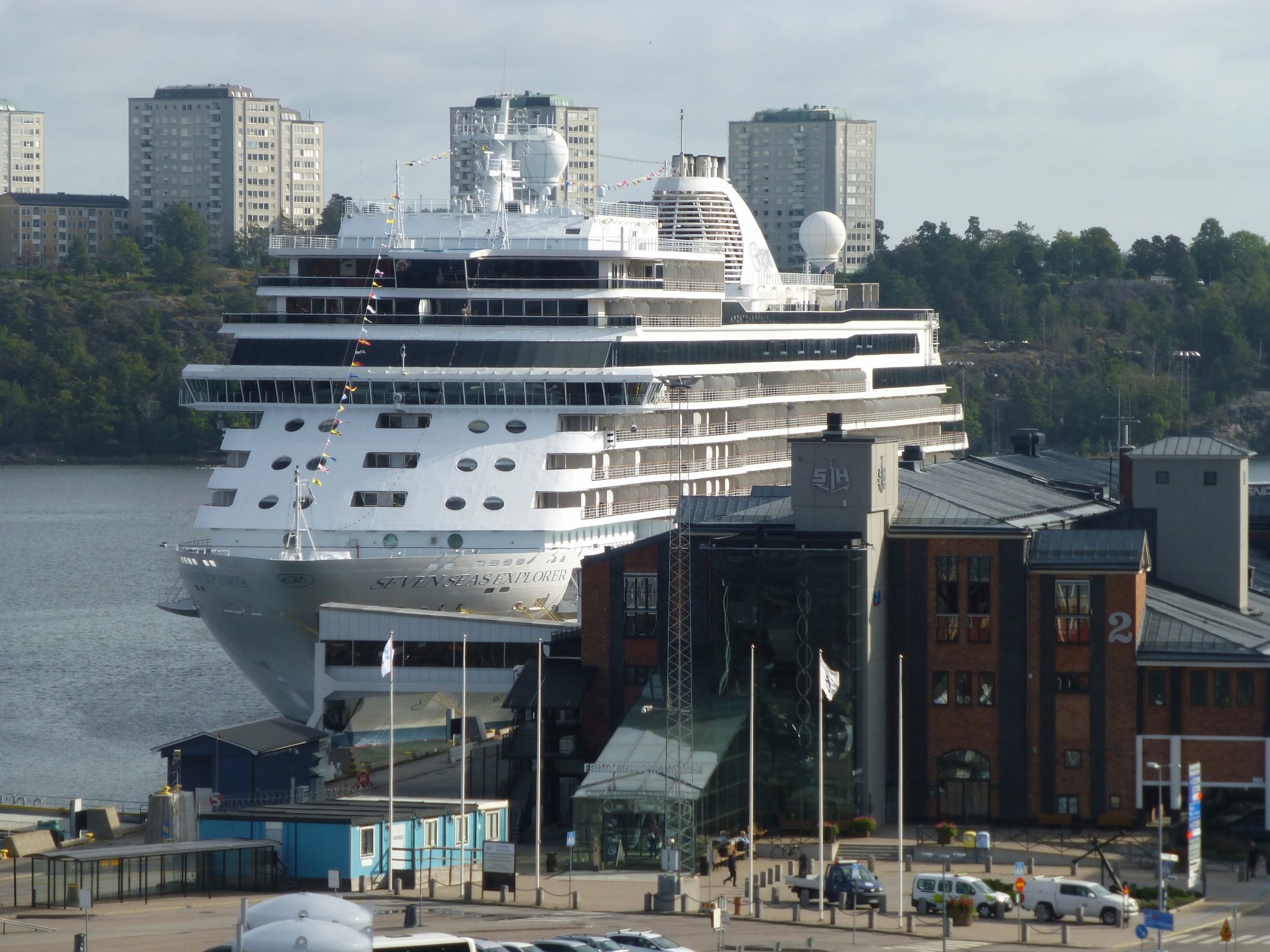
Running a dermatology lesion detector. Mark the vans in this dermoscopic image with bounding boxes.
[911,873,1014,919]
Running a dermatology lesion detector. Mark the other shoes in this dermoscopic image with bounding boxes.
[722,880,738,887]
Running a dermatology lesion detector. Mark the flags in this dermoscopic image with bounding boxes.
[819,657,840,702]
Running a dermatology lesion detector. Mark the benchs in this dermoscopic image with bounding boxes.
[705,842,749,871]
[746,822,768,842]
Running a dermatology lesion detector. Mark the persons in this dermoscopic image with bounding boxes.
[724,850,738,887]
[1265,846,1270,878]
[643,820,660,859]
[1247,841,1260,881]
[738,830,758,860]
[714,830,729,847]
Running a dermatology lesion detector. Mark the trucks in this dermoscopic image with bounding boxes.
[787,860,886,909]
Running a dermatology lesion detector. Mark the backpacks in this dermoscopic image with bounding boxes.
[647,832,655,840]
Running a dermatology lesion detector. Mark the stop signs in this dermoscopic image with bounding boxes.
[1016,878,1025,892]
[1124,886,1129,895]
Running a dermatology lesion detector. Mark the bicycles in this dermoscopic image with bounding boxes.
[770,838,803,862]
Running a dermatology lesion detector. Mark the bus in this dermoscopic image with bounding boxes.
[372,933,477,952]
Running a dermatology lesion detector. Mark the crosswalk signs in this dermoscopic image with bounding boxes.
[567,832,575,846]
[1014,862,1024,876]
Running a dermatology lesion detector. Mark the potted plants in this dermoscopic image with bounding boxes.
[848,816,878,838]
[935,821,959,846]
[939,888,980,926]
[815,821,839,844]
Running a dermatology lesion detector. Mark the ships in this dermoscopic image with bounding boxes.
[153,89,969,723]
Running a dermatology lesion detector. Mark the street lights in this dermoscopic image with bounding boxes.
[1173,349,1202,438]
[1146,761,1183,952]
[947,361,976,458]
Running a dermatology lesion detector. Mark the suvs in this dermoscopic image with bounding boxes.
[1020,875,1139,925]
[471,928,693,952]
